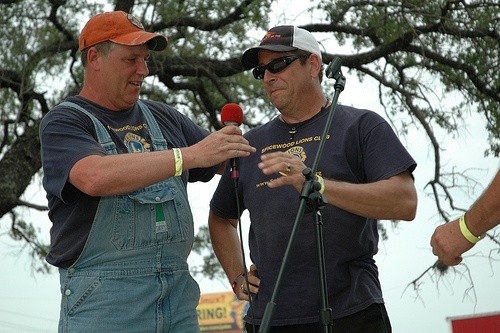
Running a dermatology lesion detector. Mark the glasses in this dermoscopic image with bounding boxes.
[252,53,303,80]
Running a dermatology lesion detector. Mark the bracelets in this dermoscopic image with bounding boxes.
[459,213,486,243]
[315,173,325,194]
[232,270,249,290]
[172,148,182,176]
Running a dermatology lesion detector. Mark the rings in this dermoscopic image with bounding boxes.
[240,283,245,290]
[285,162,293,175]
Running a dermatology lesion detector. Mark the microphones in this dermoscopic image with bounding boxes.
[221,104,243,188]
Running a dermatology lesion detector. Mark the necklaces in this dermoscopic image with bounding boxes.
[282,97,329,142]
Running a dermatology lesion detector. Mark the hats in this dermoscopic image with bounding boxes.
[241,25,322,71]
[79,11,167,52]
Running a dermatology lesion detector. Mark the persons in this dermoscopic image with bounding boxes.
[431,169,500,266]
[39,11,256,333]
[208,26,417,333]
